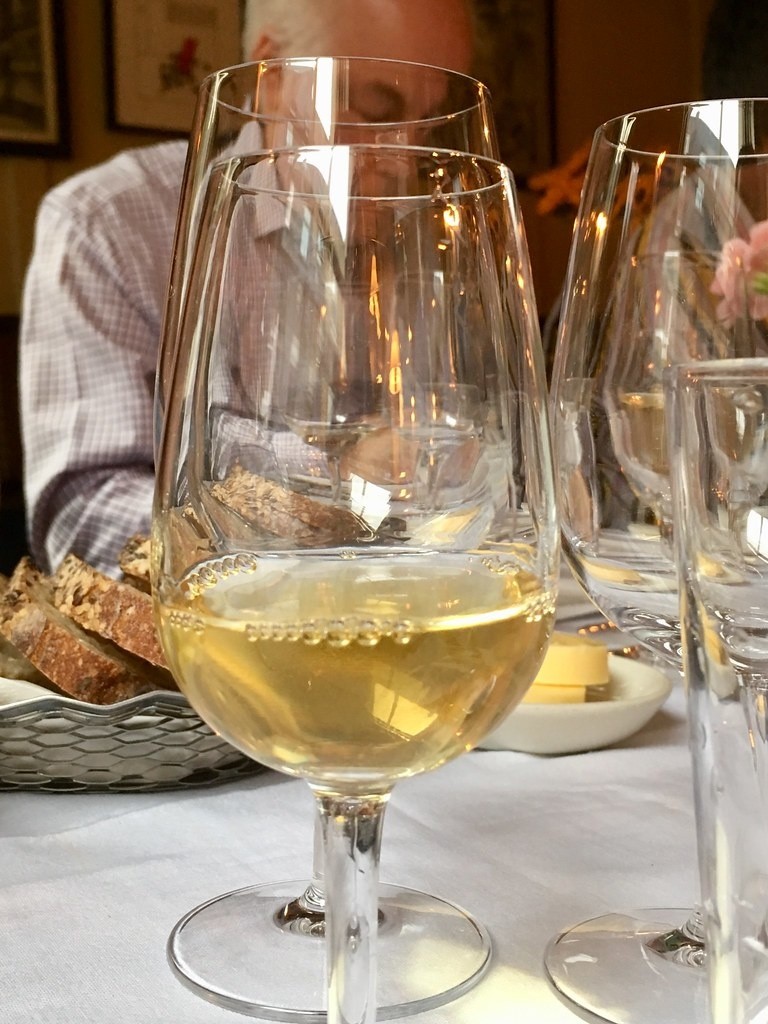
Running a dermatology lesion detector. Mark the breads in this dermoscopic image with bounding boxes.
[0,463,363,713]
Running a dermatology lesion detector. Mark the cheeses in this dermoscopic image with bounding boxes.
[517,632,609,703]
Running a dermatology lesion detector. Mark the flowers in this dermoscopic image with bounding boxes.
[709,217,768,329]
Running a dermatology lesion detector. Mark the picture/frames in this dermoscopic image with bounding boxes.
[0,0,73,158]
[451,0,562,188]
[103,0,248,137]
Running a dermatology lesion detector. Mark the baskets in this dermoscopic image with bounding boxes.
[0,689,273,793]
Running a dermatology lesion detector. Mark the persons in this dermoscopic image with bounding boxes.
[19,0,480,575]
[638,96,767,349]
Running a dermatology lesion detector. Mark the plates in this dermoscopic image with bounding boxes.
[473,652,666,751]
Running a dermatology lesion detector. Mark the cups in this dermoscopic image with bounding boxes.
[663,352,768,1024]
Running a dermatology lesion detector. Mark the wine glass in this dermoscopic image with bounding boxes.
[169,53,498,1015]
[542,93,768,1023]
[154,145,560,1024]
